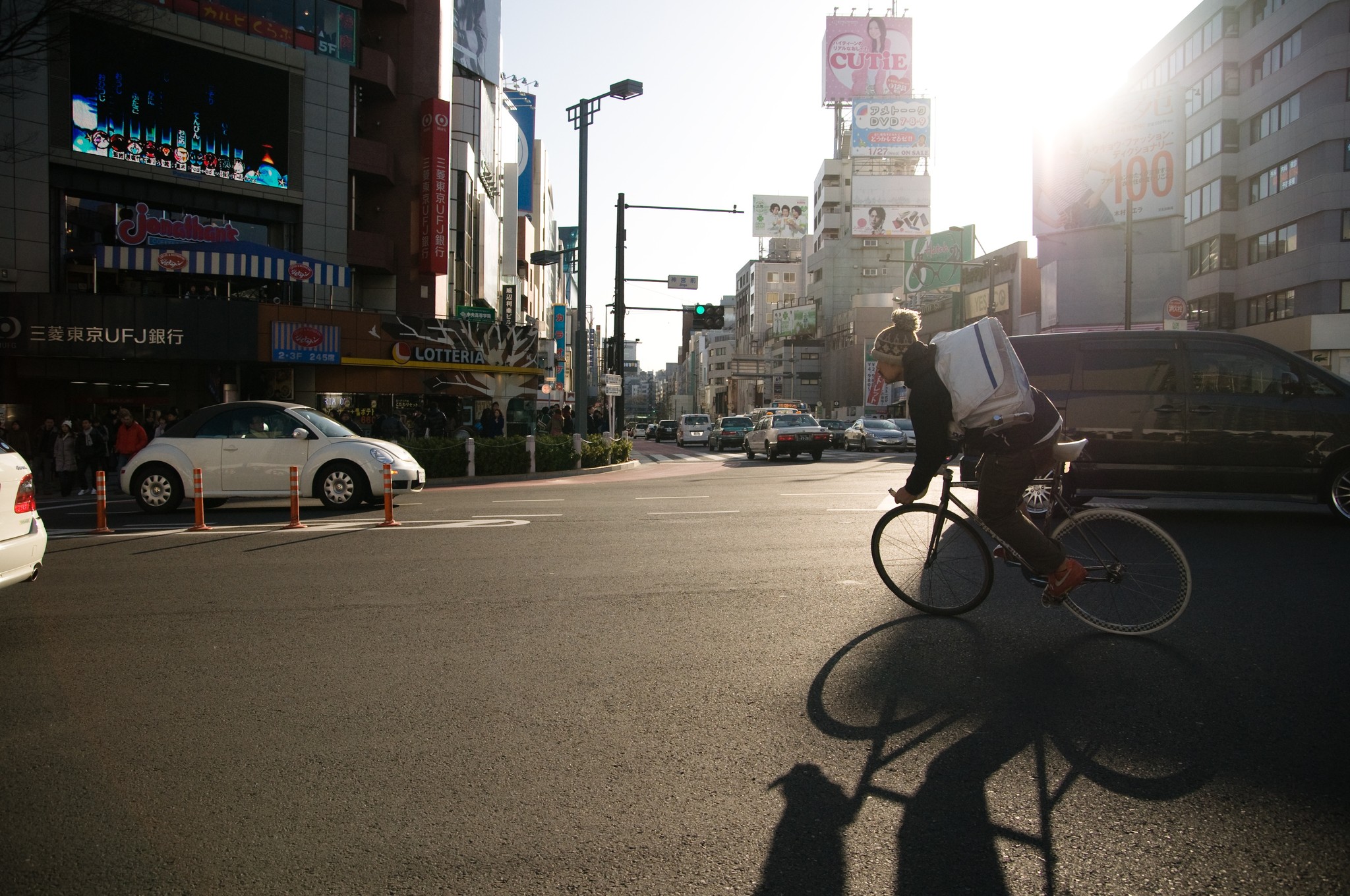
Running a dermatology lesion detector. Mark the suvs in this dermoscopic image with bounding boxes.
[624,421,649,439]
[958,327,1350,539]
[653,419,679,442]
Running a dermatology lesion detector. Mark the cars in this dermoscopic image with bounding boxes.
[674,413,712,448]
[0,441,49,589]
[119,400,428,516]
[887,418,917,451]
[742,411,831,463]
[843,418,908,453]
[707,416,756,453]
[644,424,659,441]
[814,419,848,450]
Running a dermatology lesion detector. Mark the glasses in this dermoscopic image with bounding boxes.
[253,420,263,424]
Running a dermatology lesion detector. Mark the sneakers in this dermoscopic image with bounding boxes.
[91,489,96,494]
[994,545,1005,559]
[1042,558,1088,604]
[78,488,89,495]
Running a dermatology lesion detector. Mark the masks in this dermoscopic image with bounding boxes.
[124,420,131,426]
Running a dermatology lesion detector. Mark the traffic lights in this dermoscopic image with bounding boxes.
[647,414,652,417]
[693,305,725,330]
[653,410,659,413]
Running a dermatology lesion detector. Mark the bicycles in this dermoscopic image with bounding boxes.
[871,438,1193,638]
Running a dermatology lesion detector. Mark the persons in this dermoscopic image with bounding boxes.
[329,410,363,436]
[371,401,448,443]
[871,308,1088,605]
[852,17,891,95]
[789,311,793,333]
[912,134,928,147]
[873,415,891,419]
[767,203,807,238]
[718,414,723,418]
[480,402,504,438]
[865,207,886,235]
[0,407,184,495]
[453,0,488,77]
[296,23,325,38]
[774,313,782,335]
[537,404,609,436]
[1033,153,1115,231]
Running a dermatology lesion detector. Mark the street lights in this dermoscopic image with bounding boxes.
[1112,198,1143,331]
[949,226,965,327]
[603,304,615,432]
[751,340,758,409]
[528,78,644,440]
[693,373,697,414]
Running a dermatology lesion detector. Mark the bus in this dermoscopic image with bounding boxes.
[735,399,812,429]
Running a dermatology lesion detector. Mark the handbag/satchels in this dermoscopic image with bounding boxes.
[537,415,546,430]
[929,316,1036,435]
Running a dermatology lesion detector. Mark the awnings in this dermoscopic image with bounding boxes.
[891,400,906,404]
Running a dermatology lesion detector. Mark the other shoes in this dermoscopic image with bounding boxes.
[44,491,52,495]
[115,484,121,489]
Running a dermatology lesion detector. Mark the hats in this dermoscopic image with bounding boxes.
[870,307,923,363]
[62,420,72,428]
